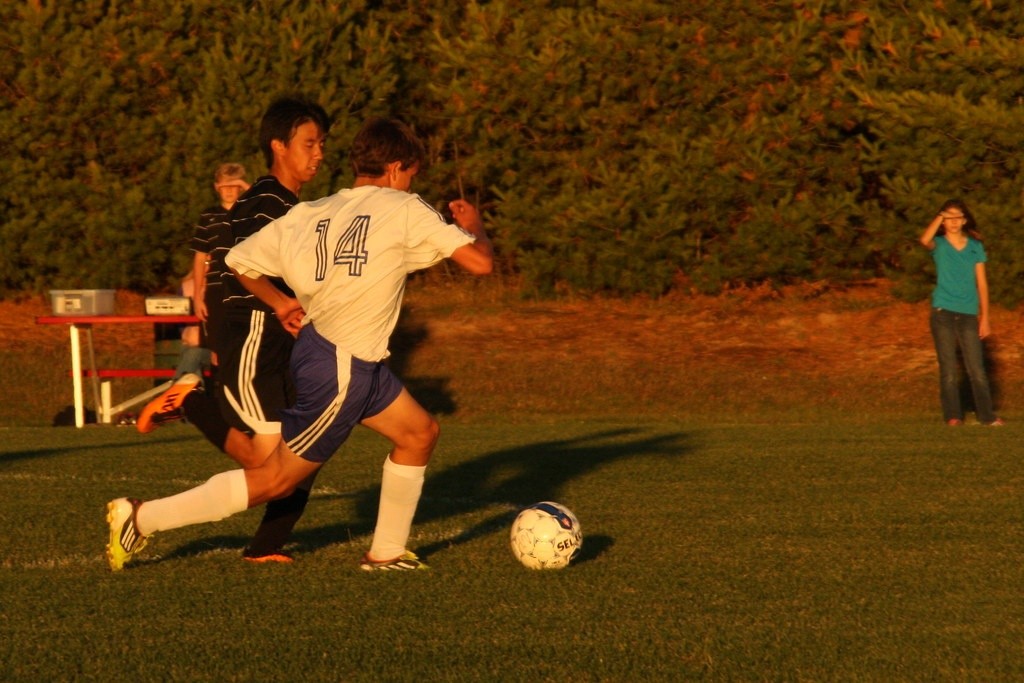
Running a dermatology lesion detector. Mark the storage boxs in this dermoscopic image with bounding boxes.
[145,297,193,315]
[48,288,116,316]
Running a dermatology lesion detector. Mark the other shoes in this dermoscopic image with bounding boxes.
[993,418,1002,425]
[948,419,963,426]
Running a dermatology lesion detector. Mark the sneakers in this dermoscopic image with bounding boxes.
[243,547,297,564]
[137,374,204,434]
[106,497,148,573]
[361,551,430,573]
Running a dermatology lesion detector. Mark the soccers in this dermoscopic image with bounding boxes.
[506,500,585,574]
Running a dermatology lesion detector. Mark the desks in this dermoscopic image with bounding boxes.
[35,315,203,429]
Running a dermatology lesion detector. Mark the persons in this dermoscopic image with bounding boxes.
[106,96,493,572]
[920,199,1007,427]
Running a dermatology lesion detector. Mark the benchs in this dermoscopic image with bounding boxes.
[68,369,212,424]
